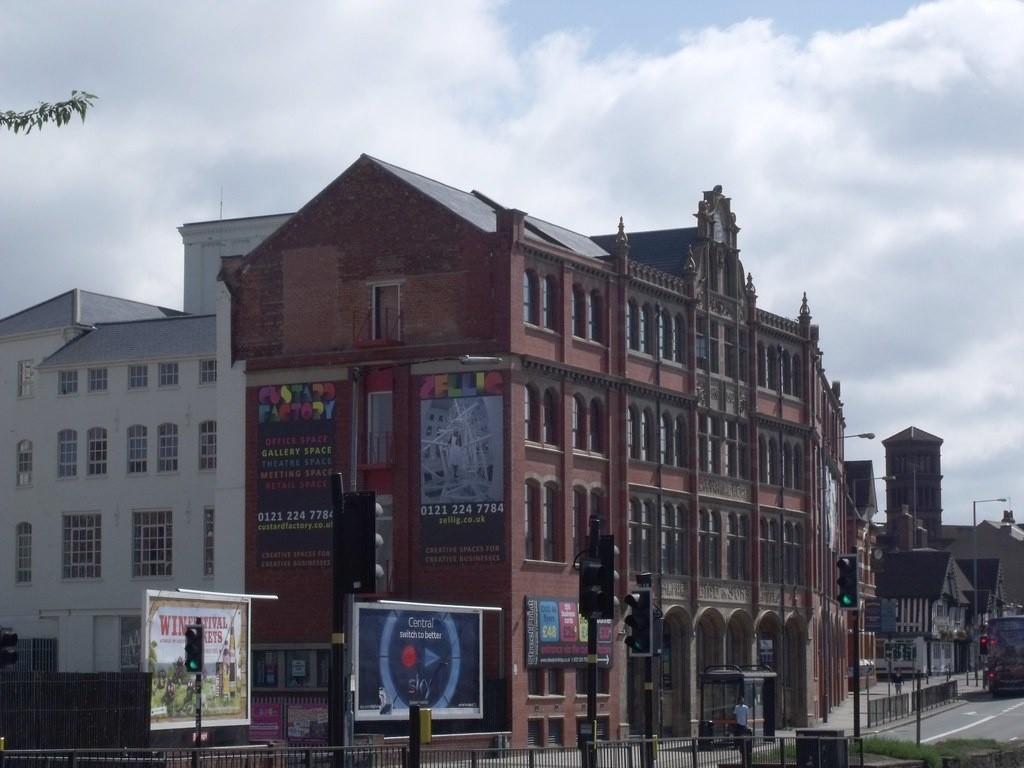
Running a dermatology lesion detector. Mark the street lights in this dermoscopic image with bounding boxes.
[819,433,876,723]
[851,476,896,549]
[972,498,1008,669]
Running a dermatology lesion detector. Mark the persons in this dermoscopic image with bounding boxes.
[892,668,904,695]
[733,697,749,750]
[447,429,462,478]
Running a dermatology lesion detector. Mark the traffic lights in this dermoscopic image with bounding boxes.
[340,490,385,593]
[624,588,655,658]
[578,559,612,620]
[0,626,19,666]
[184,624,203,672]
[980,636,987,655]
[587,534,619,620]
[836,555,858,608]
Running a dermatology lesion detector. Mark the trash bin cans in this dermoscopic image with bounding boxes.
[795,729,849,768]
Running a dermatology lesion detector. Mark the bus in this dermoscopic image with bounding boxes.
[989,616,1024,699]
